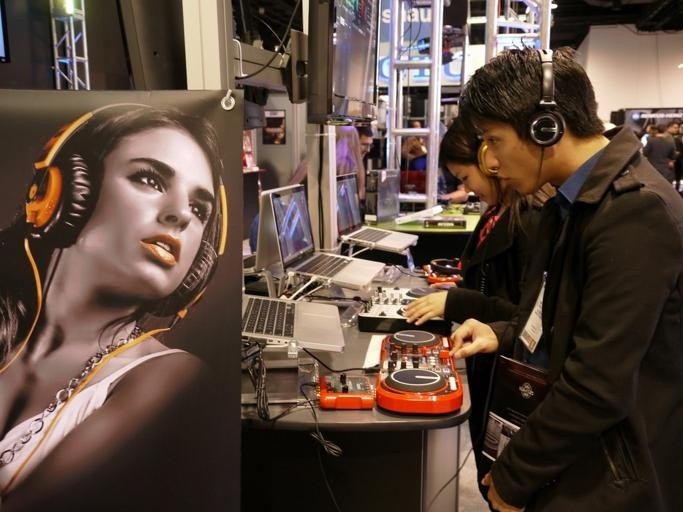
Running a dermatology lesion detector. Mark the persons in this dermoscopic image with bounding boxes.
[445,44,683,512]
[640,121,683,194]
[0,101,227,511]
[403,116,557,376]
[358,103,470,212]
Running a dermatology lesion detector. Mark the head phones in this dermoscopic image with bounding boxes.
[477,138,500,177]
[529,49,565,147]
[23,103,229,318]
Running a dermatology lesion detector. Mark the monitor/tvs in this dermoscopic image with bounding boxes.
[307,0,381,125]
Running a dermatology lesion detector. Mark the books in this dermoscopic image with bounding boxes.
[480,355,560,464]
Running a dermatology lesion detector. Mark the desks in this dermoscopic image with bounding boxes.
[238,266,471,512]
[368,201,480,259]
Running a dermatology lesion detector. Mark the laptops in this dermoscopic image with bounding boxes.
[272,170,418,290]
[242,292,347,353]
[244,181,301,276]
